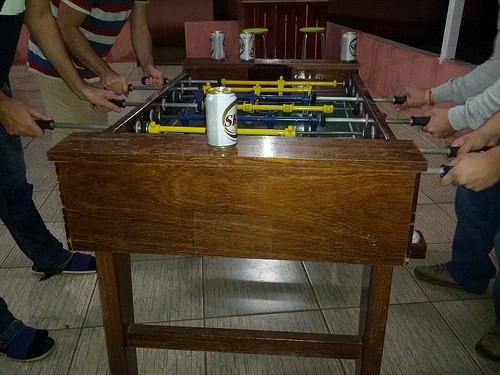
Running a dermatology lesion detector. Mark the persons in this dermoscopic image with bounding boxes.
[397,8,500,363]
[0,0,127,361]
[26,0,164,148]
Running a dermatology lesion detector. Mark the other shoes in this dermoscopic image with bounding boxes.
[414,262,480,294]
[475,323,500,359]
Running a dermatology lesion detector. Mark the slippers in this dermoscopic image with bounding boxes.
[0,326,55,361]
[32,251,98,274]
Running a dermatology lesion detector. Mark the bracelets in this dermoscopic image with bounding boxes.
[424,89,436,106]
